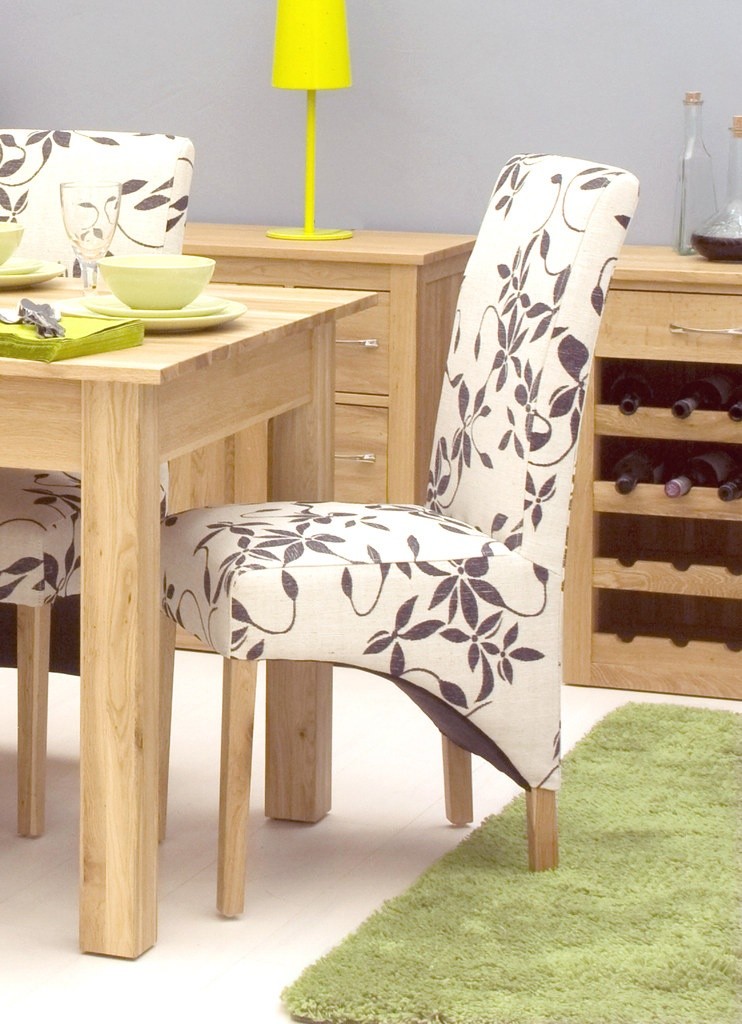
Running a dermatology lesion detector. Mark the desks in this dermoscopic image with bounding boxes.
[1,278,382,958]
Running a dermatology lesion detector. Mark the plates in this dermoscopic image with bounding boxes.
[0,270,59,287]
[87,299,225,317]
[0,262,40,274]
[62,299,247,331]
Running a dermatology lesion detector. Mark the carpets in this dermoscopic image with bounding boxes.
[274,701,742,1024]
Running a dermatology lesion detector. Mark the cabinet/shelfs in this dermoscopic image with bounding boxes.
[563,245,742,703]
[183,222,476,507]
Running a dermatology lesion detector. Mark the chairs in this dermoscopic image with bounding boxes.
[160,152,640,920]
[0,126,196,838]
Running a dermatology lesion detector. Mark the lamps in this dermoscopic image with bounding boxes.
[264,1,357,241]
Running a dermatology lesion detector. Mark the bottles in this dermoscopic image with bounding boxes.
[690,116,742,260]
[610,367,655,415]
[719,462,742,501]
[678,91,718,255]
[727,383,742,422]
[611,447,666,494]
[614,530,742,651]
[672,372,733,418]
[665,450,736,498]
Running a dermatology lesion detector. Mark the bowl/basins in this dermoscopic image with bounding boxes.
[0,222,24,266]
[96,254,216,310]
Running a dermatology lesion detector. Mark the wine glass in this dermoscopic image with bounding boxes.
[60,181,121,299]
